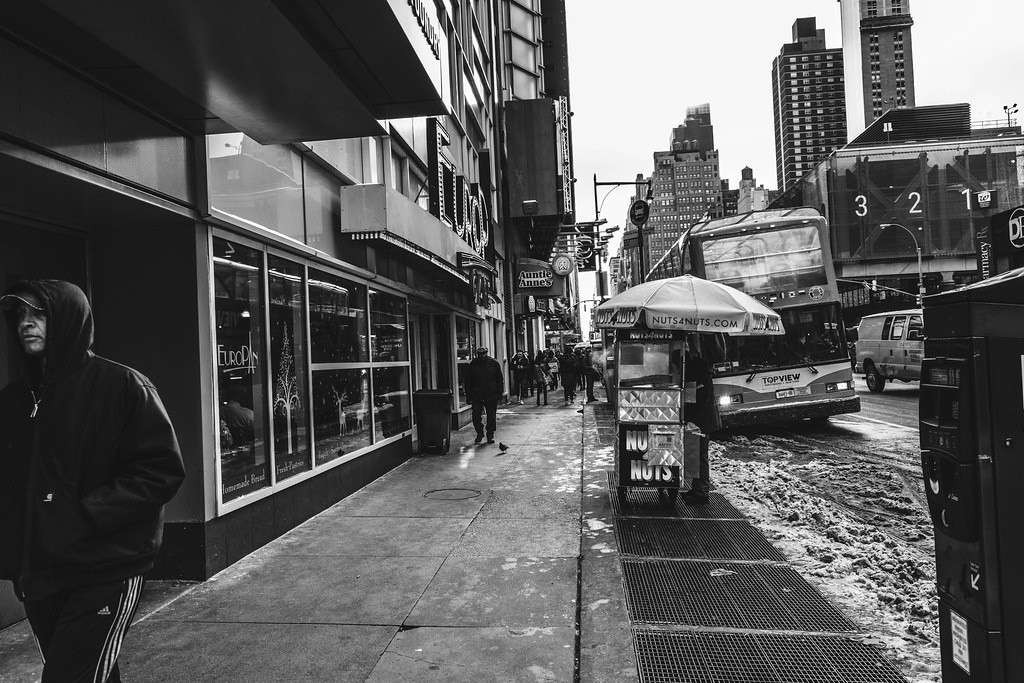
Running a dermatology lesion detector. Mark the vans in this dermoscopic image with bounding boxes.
[855,309,926,393]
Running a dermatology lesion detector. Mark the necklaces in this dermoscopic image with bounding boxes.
[31,392,42,418]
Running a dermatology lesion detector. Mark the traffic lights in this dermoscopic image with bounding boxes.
[915,293,922,305]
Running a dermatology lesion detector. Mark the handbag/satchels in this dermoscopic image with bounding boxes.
[544,374,552,384]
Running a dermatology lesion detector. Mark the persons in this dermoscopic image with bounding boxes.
[511,345,599,406]
[466,348,505,443]
[220,384,254,446]
[672,349,714,503]
[0,278,187,682]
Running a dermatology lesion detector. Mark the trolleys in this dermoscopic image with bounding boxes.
[614,329,687,503]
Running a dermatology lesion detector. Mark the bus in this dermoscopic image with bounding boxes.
[214,256,350,438]
[645,206,863,437]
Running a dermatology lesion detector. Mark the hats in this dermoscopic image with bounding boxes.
[0,288,47,312]
[547,350,554,355]
[475,346,488,354]
[564,346,573,354]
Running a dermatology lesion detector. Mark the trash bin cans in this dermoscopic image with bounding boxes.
[377,390,409,438]
[412,388,453,453]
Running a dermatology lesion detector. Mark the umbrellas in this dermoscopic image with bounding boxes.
[594,273,785,488]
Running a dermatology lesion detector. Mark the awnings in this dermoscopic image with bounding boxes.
[517,254,571,297]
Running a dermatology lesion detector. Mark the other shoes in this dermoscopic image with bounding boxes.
[518,396,520,403]
[487,436,495,443]
[475,432,484,442]
[565,401,571,406]
[571,401,574,403]
[592,396,598,401]
[520,400,524,405]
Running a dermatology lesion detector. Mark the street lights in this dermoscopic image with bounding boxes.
[593,180,653,304]
[881,223,925,309]
